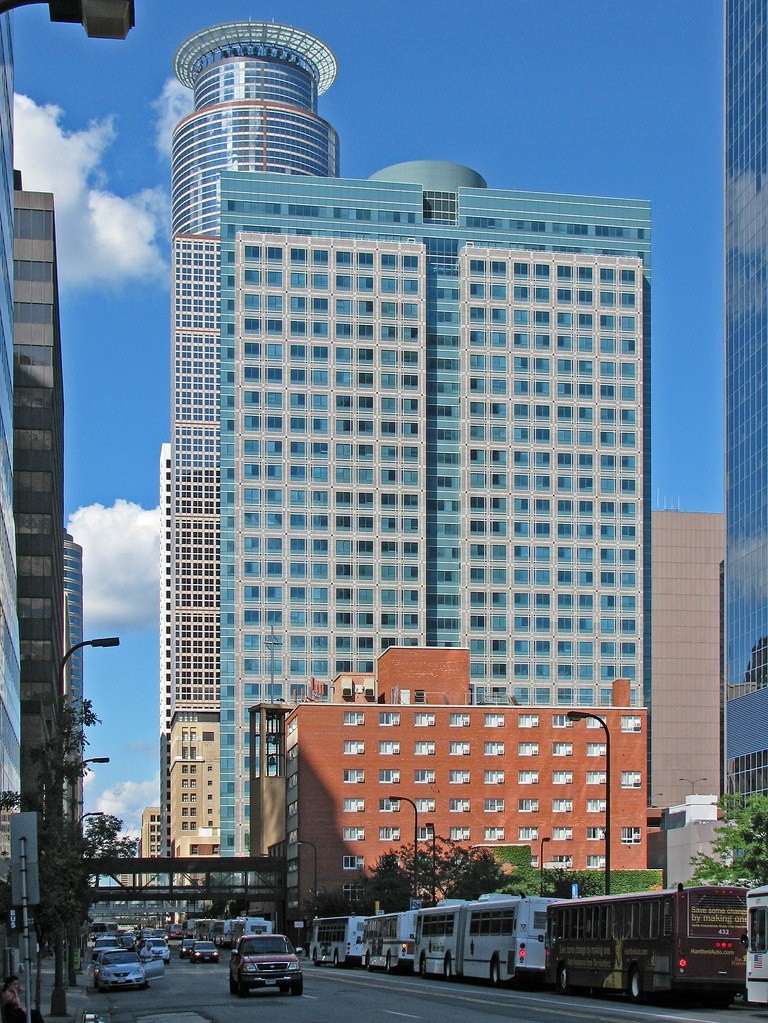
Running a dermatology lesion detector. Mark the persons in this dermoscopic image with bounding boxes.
[1,976,46,1023]
[356,936,364,952]
[139,942,153,988]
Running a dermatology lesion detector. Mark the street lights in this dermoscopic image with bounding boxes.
[565,711,613,897]
[425,822,437,907]
[539,837,551,898]
[76,811,104,854]
[66,757,111,988]
[679,778,710,795]
[48,636,122,1018]
[387,795,419,899]
[295,840,318,916]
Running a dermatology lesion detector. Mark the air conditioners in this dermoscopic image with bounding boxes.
[356,863,364,868]
[532,862,538,868]
[567,862,573,868]
[356,718,644,841]
[599,861,605,868]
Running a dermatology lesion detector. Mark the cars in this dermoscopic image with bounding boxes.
[301,915,372,969]
[190,940,219,964]
[144,938,172,965]
[88,921,165,992]
[177,938,199,958]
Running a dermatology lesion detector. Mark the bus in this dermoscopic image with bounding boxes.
[355,908,420,976]
[408,892,570,990]
[537,882,750,1011]
[162,917,273,949]
[740,885,768,1007]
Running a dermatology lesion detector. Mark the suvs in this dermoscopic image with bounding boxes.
[229,934,304,998]
